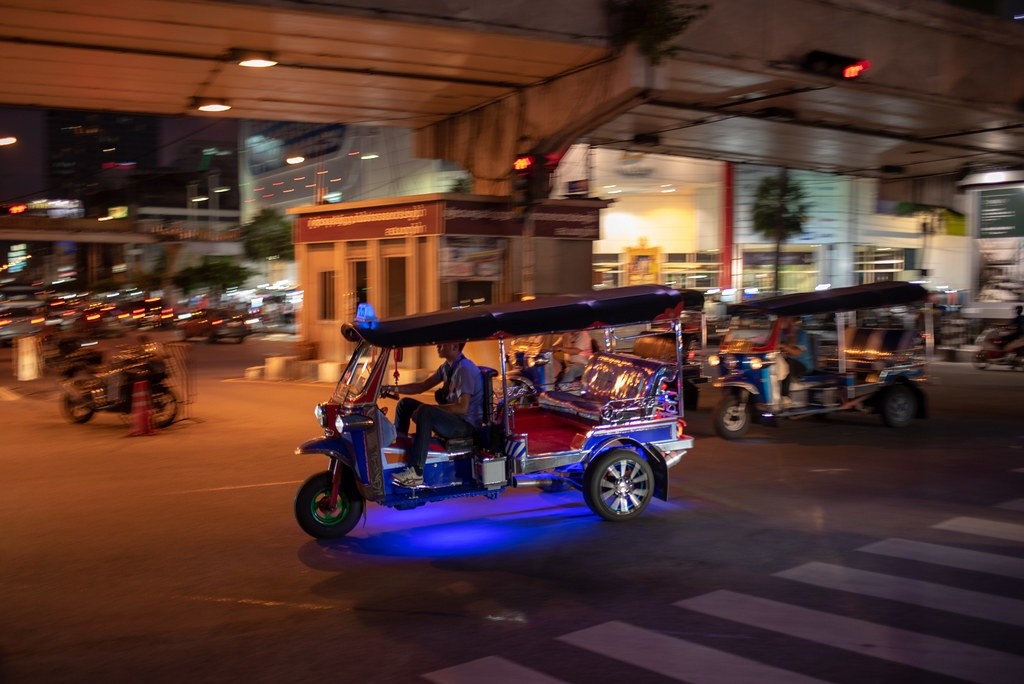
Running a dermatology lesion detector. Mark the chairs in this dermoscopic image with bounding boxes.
[795,333,819,380]
[434,366,499,452]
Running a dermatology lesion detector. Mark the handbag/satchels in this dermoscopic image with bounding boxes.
[435,387,447,404]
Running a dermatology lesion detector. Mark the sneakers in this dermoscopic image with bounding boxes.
[392,466,424,486]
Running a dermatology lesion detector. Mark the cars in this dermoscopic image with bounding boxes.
[175,307,249,344]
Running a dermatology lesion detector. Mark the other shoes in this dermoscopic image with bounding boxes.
[780,397,793,411]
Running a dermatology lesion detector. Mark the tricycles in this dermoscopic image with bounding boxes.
[292,284,705,540]
[712,280,931,440]
[495,326,634,424]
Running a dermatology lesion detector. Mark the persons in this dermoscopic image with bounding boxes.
[993,305,1024,349]
[779,317,815,404]
[382,341,485,486]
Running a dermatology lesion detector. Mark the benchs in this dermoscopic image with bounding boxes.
[631,329,696,366]
[534,353,675,426]
[820,326,916,368]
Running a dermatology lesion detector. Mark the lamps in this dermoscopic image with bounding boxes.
[237,52,279,67]
[197,97,232,111]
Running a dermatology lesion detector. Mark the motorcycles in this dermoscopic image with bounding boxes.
[46,334,176,428]
